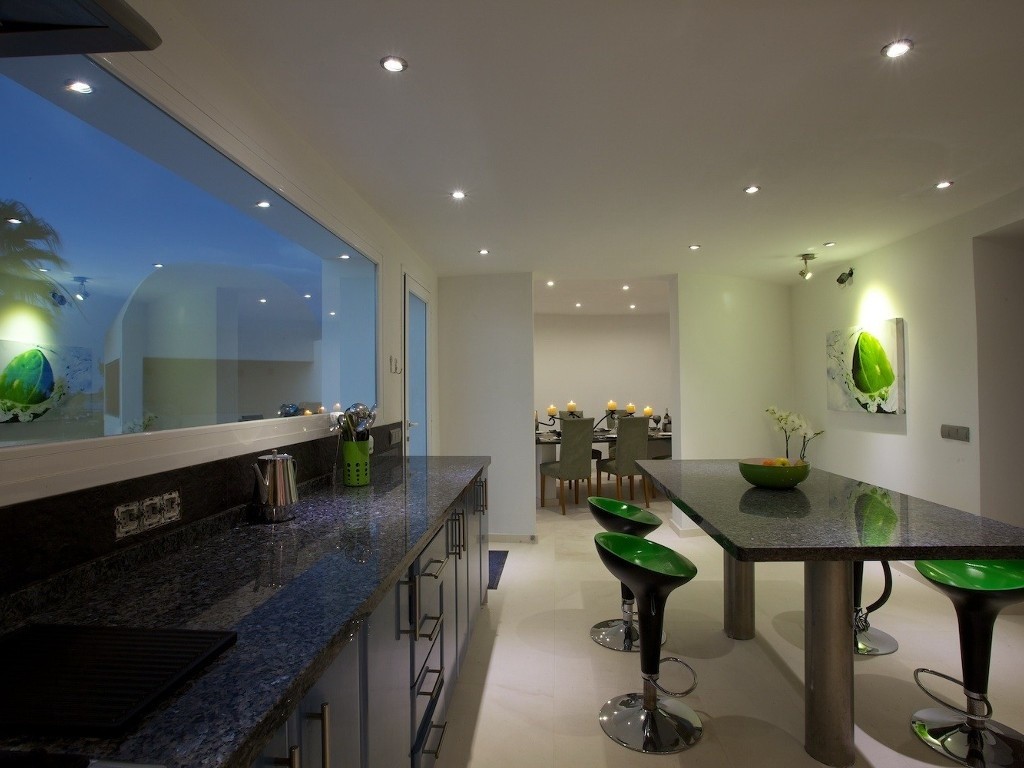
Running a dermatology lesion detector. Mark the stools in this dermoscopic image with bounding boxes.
[585,495,708,756]
[909,561,1024,768]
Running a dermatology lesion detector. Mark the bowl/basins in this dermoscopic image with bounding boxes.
[738,457,811,489]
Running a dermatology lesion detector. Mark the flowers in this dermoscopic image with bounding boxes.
[763,405,821,459]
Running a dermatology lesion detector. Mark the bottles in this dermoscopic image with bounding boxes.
[664,408,670,432]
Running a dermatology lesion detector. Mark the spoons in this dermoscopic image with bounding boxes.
[337,402,376,440]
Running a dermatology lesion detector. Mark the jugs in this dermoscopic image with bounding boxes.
[250,448,301,524]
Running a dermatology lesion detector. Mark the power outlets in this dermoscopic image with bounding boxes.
[112,489,183,544]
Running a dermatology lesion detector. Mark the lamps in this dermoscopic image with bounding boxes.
[797,252,821,282]
[72,276,92,301]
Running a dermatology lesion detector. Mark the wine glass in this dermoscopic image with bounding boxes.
[653,415,661,431]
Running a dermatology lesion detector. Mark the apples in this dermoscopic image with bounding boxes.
[763,457,790,467]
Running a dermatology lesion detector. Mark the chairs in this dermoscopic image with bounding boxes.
[539,408,650,516]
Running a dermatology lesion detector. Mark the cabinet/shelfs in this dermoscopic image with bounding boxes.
[250,470,491,768]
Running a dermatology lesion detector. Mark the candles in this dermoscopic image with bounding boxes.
[547,404,557,415]
[567,400,576,411]
[626,403,636,412]
[607,400,617,410]
[643,405,653,417]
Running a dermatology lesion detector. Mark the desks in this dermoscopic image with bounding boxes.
[633,459,1024,768]
[538,428,672,507]
